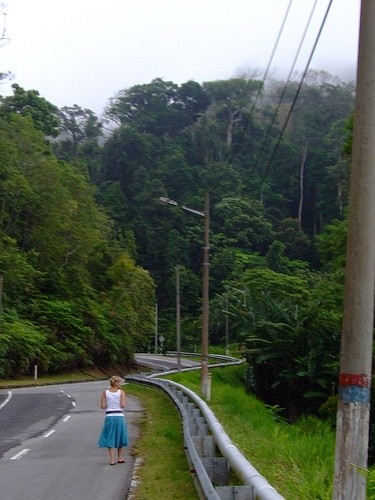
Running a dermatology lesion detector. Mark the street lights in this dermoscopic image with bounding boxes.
[224,284,247,308]
[158,191,210,396]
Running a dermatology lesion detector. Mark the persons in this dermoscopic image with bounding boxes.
[99,376,127,465]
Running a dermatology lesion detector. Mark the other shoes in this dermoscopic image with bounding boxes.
[117,461,124,463]
[110,462,115,465]
